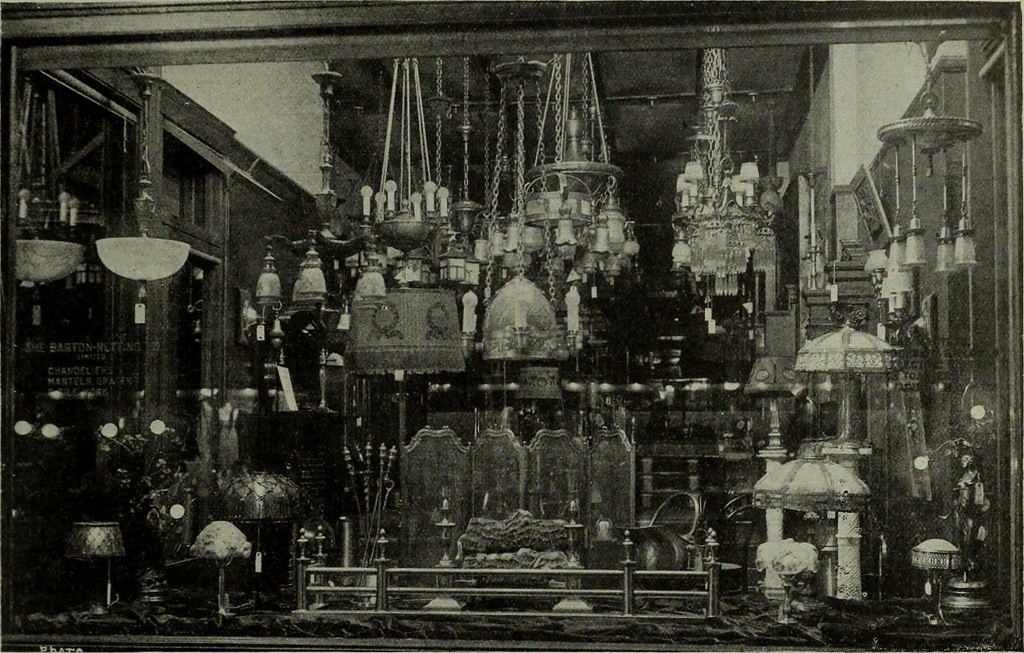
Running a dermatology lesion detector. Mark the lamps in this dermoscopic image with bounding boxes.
[23,40,1004,621]
